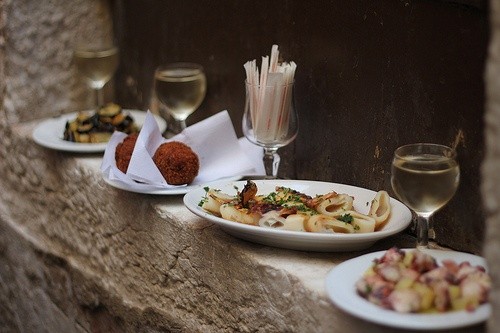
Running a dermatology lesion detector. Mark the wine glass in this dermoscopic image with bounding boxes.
[155,62,206,133]
[74,38,121,107]
[391,143,459,249]
[241,72,299,180]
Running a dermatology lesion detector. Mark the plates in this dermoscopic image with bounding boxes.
[101,170,244,194]
[326,249,492,330]
[32,110,167,153]
[184,179,413,252]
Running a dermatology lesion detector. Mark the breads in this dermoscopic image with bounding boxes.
[115,133,139,173]
[152,142,199,185]
